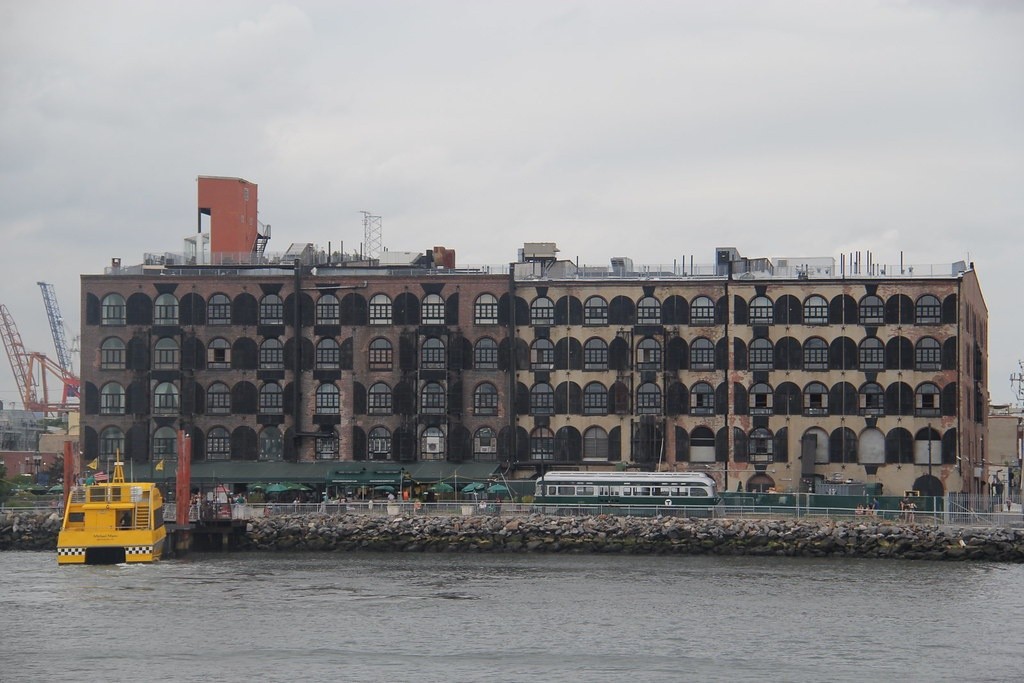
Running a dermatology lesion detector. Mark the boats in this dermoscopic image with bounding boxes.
[53,448,168,568]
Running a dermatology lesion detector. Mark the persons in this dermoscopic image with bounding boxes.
[479,496,503,516]
[387,493,395,501]
[414,499,422,512]
[293,497,301,504]
[190,492,247,506]
[855,497,918,527]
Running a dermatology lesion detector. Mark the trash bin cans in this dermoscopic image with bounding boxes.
[461,505,475,515]
[387,506,402,515]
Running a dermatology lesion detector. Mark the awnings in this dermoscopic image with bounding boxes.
[108,460,502,482]
[373,485,394,492]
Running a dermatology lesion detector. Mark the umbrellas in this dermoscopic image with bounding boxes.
[247,480,266,494]
[461,482,485,506]
[47,485,64,499]
[428,482,454,499]
[488,483,508,497]
[267,481,313,500]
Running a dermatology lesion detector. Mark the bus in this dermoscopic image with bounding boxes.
[529,468,721,518]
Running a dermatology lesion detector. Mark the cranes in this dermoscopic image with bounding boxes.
[0,282,81,417]
